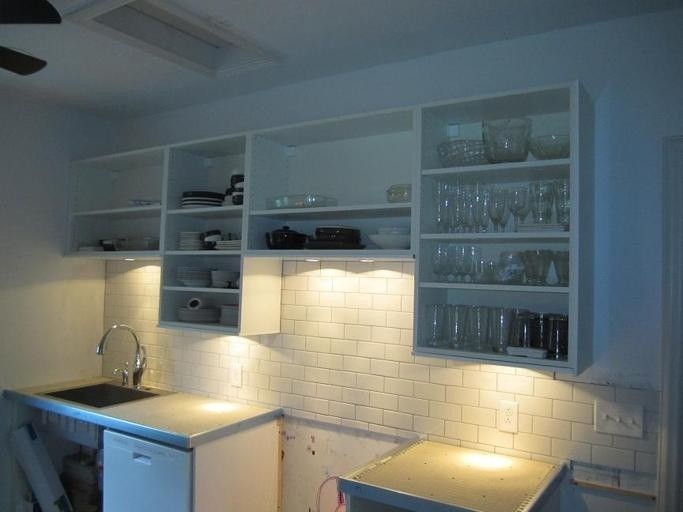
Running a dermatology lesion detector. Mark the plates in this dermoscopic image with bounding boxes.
[177,266,211,288]
[176,229,242,250]
[180,191,226,208]
[124,199,161,207]
[176,304,239,326]
[303,227,366,250]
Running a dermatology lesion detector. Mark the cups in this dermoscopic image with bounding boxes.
[424,303,568,361]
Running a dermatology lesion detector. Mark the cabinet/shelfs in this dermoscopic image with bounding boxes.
[61,79,597,378]
[103,415,282,512]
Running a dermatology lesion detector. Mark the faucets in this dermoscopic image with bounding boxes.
[96,323,149,389]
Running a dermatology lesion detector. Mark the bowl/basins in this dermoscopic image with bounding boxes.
[223,174,244,206]
[438,115,570,167]
[368,227,411,249]
[210,271,239,282]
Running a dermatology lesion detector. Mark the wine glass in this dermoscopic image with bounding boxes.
[431,181,569,285]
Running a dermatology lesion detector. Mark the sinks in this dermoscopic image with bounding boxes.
[42,382,161,411]
